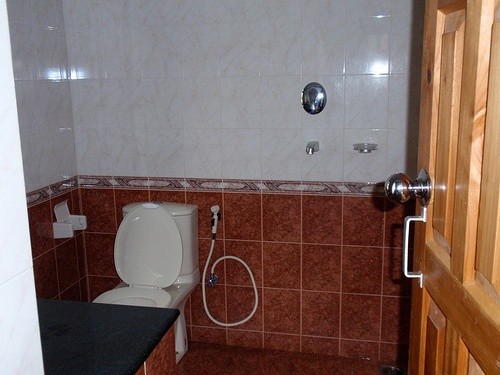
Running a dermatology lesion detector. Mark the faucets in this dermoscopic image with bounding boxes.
[305,140,319,154]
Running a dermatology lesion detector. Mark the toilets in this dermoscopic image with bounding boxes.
[93,201,201,365]
